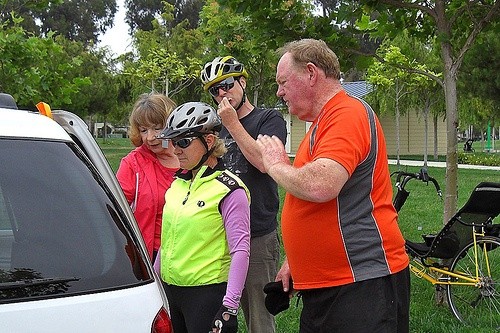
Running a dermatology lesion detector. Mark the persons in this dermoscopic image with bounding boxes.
[201,56,287,333]
[150,101,251,333]
[256,37,411,333]
[115,92,180,265]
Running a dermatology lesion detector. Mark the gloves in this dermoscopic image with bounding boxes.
[210,306,238,333]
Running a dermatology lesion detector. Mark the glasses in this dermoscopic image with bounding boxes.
[172,135,208,148]
[208,76,240,97]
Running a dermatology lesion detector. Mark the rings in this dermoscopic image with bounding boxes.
[217,106,221,109]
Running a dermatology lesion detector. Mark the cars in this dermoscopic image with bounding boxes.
[0,93,173,333]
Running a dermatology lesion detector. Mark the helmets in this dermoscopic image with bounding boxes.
[157,101,221,139]
[200,55,248,90]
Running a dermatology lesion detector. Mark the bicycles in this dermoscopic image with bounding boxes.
[385,167,500,329]
